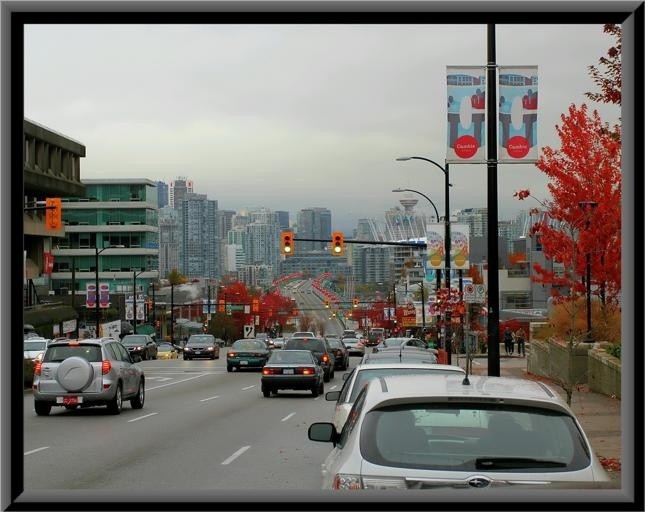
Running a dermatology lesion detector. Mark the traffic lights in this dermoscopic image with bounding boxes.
[281,232,293,256]
[332,228,345,257]
[332,232,344,256]
[281,229,297,260]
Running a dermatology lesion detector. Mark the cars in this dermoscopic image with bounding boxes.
[121,334,158,363]
[226,338,271,372]
[155,345,179,360]
[159,342,182,354]
[326,339,352,369]
[262,349,324,400]
[183,333,219,362]
[342,337,365,356]
[215,338,225,348]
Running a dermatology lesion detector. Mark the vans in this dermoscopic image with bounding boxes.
[23,336,51,364]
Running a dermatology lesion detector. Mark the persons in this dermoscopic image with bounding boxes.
[504,327,511,354]
[511,328,516,354]
[516,327,526,357]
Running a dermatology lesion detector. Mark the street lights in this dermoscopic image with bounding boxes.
[392,188,443,354]
[395,157,452,363]
[23,195,55,302]
[95,245,125,337]
[132,269,157,334]
[134,266,146,332]
[92,242,116,340]
[393,184,444,331]
[396,156,464,345]
[171,278,200,351]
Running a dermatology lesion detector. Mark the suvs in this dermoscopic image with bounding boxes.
[34,339,146,413]
[285,336,334,384]
